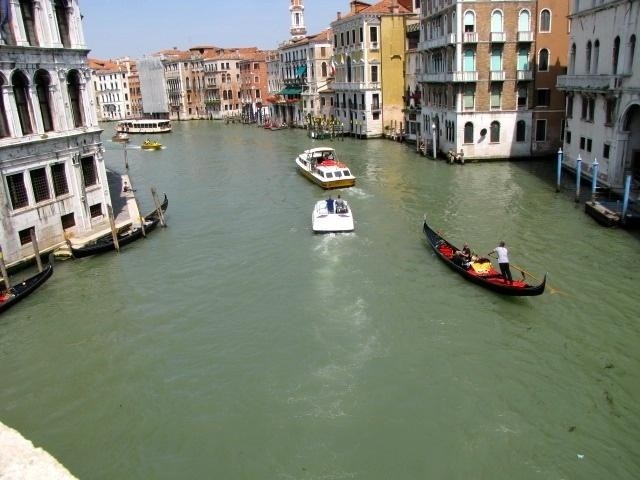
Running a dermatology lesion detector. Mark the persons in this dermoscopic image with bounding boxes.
[122,182,129,192]
[335,194,344,207]
[460,242,471,258]
[325,196,334,214]
[487,240,512,281]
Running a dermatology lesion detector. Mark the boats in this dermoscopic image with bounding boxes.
[114,119,172,134]
[311,198,354,233]
[66,193,169,260]
[296,146,356,190]
[141,141,162,150]
[112,134,130,144]
[421,214,547,297]
[0,265,54,318]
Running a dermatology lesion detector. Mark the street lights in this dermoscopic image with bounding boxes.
[431,122,437,159]
